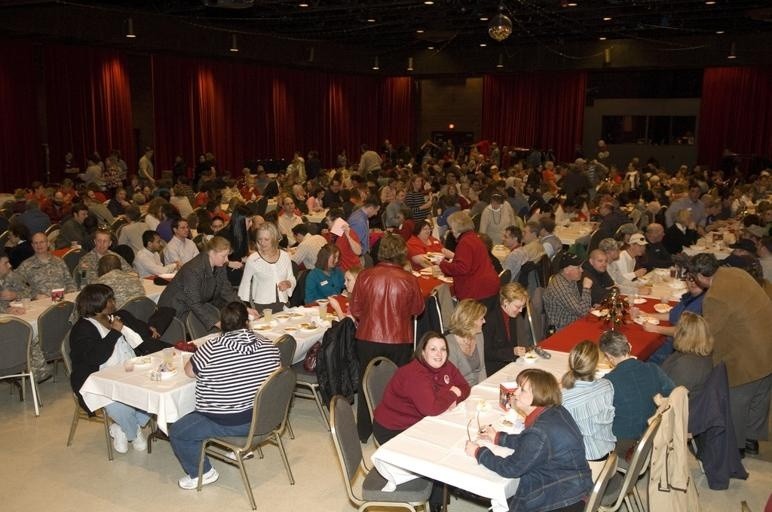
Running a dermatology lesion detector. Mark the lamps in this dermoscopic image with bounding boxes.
[407,57,414,72]
[229,36,240,53]
[372,56,380,71]
[125,16,140,40]
[496,54,507,70]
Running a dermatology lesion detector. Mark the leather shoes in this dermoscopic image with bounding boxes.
[429,502,441,512]
[743,437,759,455]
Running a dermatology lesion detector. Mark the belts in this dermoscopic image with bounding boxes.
[588,450,613,461]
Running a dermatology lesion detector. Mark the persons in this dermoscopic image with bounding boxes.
[1,139,772,512]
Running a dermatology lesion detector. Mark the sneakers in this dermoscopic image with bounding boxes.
[178,466,220,489]
[132,423,148,451]
[226,450,254,461]
[109,422,129,453]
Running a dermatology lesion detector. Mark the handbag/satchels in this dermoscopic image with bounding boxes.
[304,341,320,372]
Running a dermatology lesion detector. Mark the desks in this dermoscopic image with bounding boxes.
[370,348,614,512]
[264,196,742,360]
[79,291,355,435]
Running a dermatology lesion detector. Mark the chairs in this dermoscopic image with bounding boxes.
[584,455,617,511]
[0,198,232,281]
[686,359,738,477]
[362,355,399,425]
[61,324,157,459]
[277,334,299,366]
[294,319,356,431]
[614,388,703,512]
[600,415,662,512]
[1,271,177,347]
[196,367,294,511]
[186,303,224,342]
[1,316,41,420]
[328,393,432,512]
[37,302,75,383]
[116,297,157,324]
[159,317,186,345]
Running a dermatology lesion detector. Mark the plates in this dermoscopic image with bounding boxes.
[286,312,304,320]
[653,303,671,313]
[591,309,609,317]
[129,356,164,368]
[8,301,24,308]
[633,316,661,326]
[624,296,648,305]
[252,324,274,332]
[424,252,445,261]
[298,323,320,333]
[436,275,454,284]
[160,370,177,382]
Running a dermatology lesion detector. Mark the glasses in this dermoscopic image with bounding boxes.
[511,302,527,309]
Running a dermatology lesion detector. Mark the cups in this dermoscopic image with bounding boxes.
[431,265,441,279]
[630,307,639,320]
[124,360,134,372]
[661,296,669,305]
[319,306,327,319]
[263,309,273,320]
[627,294,634,306]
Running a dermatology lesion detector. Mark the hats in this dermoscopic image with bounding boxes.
[436,208,459,226]
[741,224,765,238]
[490,165,498,170]
[628,233,649,245]
[728,239,757,255]
[599,237,624,252]
[491,190,505,204]
[560,252,584,269]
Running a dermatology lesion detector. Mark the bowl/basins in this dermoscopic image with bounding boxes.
[523,354,538,364]
[316,299,329,306]
[282,327,297,334]
[274,315,291,322]
